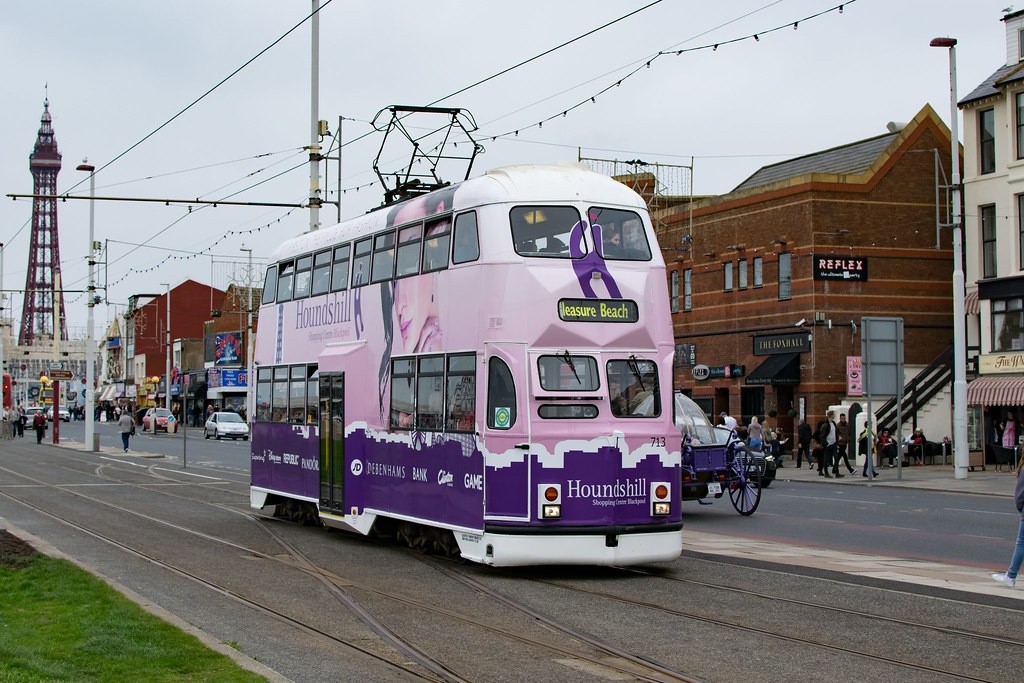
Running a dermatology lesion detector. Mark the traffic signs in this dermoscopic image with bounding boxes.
[49,369,73,380]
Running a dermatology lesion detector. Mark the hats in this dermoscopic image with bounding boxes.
[719,412,726,418]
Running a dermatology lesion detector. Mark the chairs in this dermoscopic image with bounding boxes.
[990,441,1015,471]
[876,441,951,467]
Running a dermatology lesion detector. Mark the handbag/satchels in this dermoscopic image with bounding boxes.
[130,417,135,435]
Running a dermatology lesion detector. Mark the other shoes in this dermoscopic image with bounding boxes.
[836,474,845,478]
[124,449,129,454]
[825,475,833,478]
[873,473,879,477]
[863,473,868,477]
[819,473,825,476]
[37,441,41,444]
[809,463,814,469]
[888,463,894,467]
[850,470,857,474]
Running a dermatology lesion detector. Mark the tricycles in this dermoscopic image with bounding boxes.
[676,423,762,517]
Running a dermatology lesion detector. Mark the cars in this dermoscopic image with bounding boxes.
[140,407,178,433]
[203,411,249,441]
[679,423,777,489]
[47,405,70,423]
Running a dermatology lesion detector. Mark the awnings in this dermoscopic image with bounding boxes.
[98,386,117,401]
[963,291,979,315]
[966,377,1024,407]
[745,354,800,385]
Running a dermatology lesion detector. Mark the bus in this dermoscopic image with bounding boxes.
[247,102,685,570]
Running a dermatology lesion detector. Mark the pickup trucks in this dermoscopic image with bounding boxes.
[22,406,49,430]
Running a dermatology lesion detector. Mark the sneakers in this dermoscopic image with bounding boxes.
[992,571,1015,587]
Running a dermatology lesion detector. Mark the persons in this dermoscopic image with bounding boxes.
[3,406,46,445]
[602,228,621,256]
[719,410,858,480]
[385,186,460,353]
[999,410,1021,472]
[69,401,140,423]
[858,421,879,478]
[173,404,247,428]
[610,380,624,416]
[546,239,564,253]
[429,379,448,415]
[118,410,135,453]
[877,428,898,469]
[910,428,926,465]
[990,444,1024,588]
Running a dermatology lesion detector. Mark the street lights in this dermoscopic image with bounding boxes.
[74,164,97,452]
[159,283,171,412]
[929,35,969,481]
[240,247,253,427]
